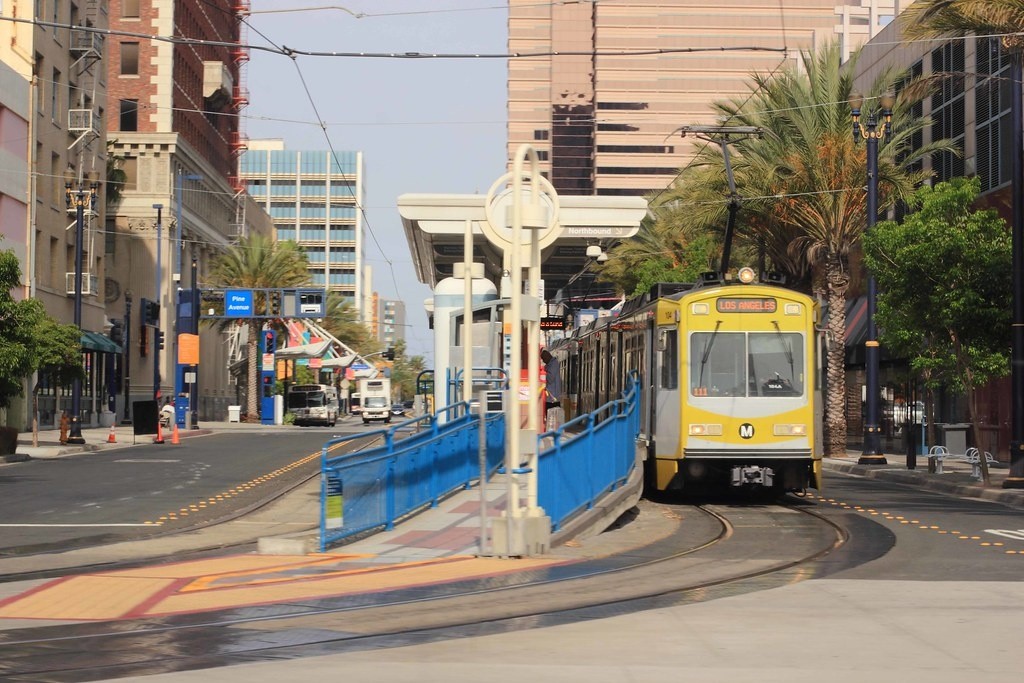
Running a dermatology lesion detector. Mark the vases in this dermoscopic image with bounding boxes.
[0,426,19,456]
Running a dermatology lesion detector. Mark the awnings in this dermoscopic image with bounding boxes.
[81,334,122,354]
[820,295,889,346]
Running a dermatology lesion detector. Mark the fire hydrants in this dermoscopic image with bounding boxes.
[57,414,70,446]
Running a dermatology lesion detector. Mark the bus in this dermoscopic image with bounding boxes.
[550,266,837,505]
[289,384,340,427]
[351,392,363,415]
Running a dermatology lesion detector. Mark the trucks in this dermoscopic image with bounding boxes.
[360,378,393,423]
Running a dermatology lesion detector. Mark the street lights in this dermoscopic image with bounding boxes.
[848,85,896,464]
[63,160,100,444]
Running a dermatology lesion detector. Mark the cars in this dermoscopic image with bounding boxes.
[391,404,406,417]
[404,400,414,408]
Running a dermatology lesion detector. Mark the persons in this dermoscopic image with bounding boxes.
[541,351,561,415]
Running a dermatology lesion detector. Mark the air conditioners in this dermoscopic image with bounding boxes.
[67,190,99,217]
[67,109,101,136]
[65,273,98,298]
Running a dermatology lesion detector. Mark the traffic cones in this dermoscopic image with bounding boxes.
[106,421,117,443]
[169,423,181,444]
[153,421,165,444]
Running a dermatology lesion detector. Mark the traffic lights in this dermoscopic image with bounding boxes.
[266,332,274,354]
[111,325,122,343]
[155,328,164,350]
[142,297,153,324]
[264,376,272,384]
